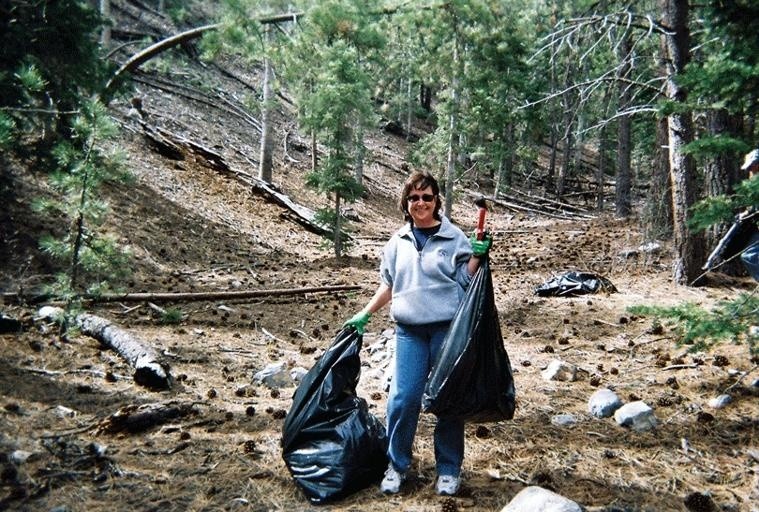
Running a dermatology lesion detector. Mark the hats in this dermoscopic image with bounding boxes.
[740,149,759,171]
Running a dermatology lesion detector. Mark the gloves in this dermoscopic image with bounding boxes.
[342,311,371,335]
[471,232,492,257]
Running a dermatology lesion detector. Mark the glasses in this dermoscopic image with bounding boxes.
[406,194,435,201]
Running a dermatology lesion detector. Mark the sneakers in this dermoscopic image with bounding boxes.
[436,474,461,495]
[380,462,407,494]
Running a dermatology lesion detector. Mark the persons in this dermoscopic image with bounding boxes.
[735,149,759,282]
[341,172,492,496]
